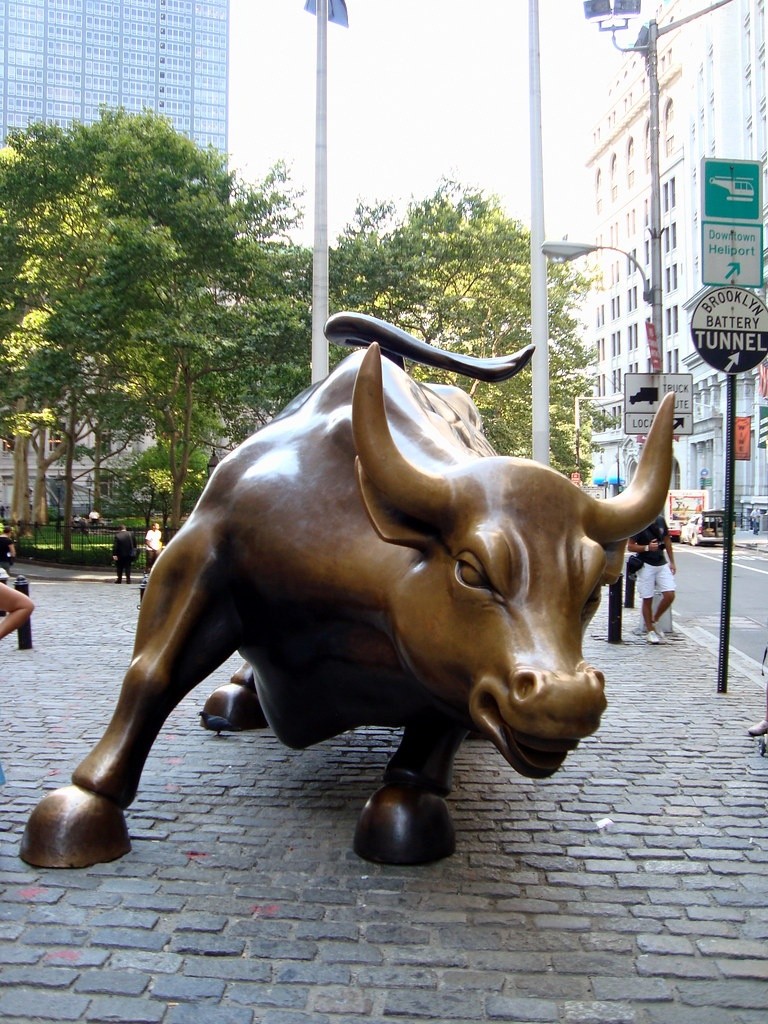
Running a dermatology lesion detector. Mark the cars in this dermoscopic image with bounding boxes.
[680,508,736,547]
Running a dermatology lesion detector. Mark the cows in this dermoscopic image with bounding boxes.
[18,311,678,870]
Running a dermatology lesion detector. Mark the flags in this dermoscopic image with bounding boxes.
[758,365,768,399]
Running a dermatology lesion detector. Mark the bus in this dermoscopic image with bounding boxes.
[663,490,709,541]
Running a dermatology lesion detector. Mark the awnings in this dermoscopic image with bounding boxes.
[594,477,621,485]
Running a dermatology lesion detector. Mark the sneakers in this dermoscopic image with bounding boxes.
[651,619,665,638]
[647,631,660,642]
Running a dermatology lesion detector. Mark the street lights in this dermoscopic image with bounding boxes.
[575,395,625,467]
[300,0,349,386]
[541,1,660,632]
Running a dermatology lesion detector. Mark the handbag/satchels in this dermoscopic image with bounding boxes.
[628,555,643,573]
[131,548,140,561]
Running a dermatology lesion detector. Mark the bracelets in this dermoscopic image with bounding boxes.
[645,545,649,551]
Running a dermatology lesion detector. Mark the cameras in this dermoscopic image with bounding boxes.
[655,538,666,552]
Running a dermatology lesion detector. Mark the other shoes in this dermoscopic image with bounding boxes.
[126,580,131,584]
[115,579,122,584]
[748,720,768,735]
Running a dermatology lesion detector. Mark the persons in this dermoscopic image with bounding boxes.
[0,526,16,575]
[74,513,88,534]
[628,514,676,644]
[88,508,100,530]
[144,523,163,578]
[0,582,34,641]
[750,506,761,535]
[112,523,137,584]
[748,682,768,736]
